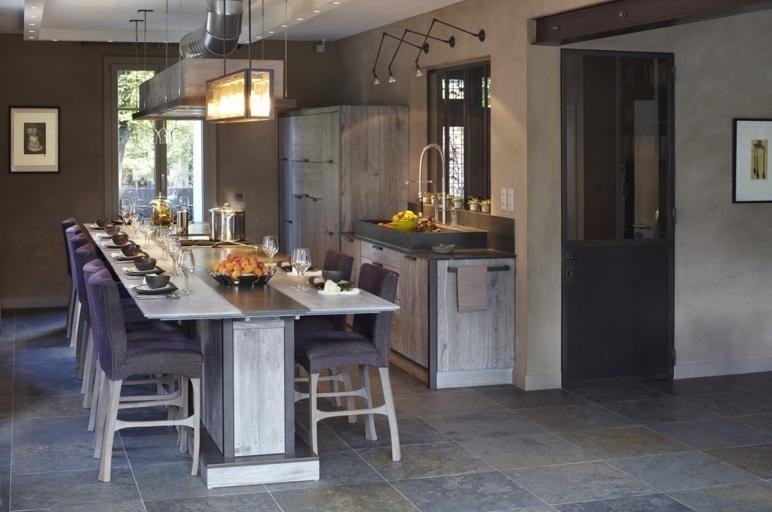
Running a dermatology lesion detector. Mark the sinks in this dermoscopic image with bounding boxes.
[355,218,465,235]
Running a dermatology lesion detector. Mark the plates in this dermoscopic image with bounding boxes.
[113,250,150,261]
[97,231,127,239]
[132,282,178,295]
[91,224,112,230]
[307,276,354,288]
[126,265,166,276]
[106,239,137,249]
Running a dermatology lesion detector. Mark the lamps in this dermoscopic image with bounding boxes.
[366,18,486,89]
[200,0,280,126]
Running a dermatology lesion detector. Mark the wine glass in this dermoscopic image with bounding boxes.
[156,226,181,277]
[262,236,279,277]
[140,217,155,250]
[180,250,197,297]
[179,196,190,210]
[291,248,312,292]
[121,200,139,230]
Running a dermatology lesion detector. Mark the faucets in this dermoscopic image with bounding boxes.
[418,142,447,226]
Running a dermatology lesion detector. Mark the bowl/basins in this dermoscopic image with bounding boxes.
[112,232,129,245]
[134,258,157,269]
[95,217,112,226]
[145,274,171,288]
[105,226,120,235]
[321,269,345,282]
[121,244,141,257]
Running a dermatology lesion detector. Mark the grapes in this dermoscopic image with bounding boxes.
[412,222,438,233]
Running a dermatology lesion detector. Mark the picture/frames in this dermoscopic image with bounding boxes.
[8,105,61,175]
[732,116,771,203]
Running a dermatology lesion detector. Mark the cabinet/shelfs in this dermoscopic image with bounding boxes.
[281,106,408,264]
[349,237,515,394]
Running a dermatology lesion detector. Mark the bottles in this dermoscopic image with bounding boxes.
[176,211,189,236]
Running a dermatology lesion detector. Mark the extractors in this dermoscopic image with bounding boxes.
[132,57,297,120]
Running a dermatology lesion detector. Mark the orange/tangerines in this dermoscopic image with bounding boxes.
[212,252,272,280]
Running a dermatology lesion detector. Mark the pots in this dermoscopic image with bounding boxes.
[207,202,247,242]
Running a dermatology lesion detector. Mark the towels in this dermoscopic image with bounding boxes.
[452,264,490,314]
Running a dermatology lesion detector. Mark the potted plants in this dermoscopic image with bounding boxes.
[419,192,492,211]
